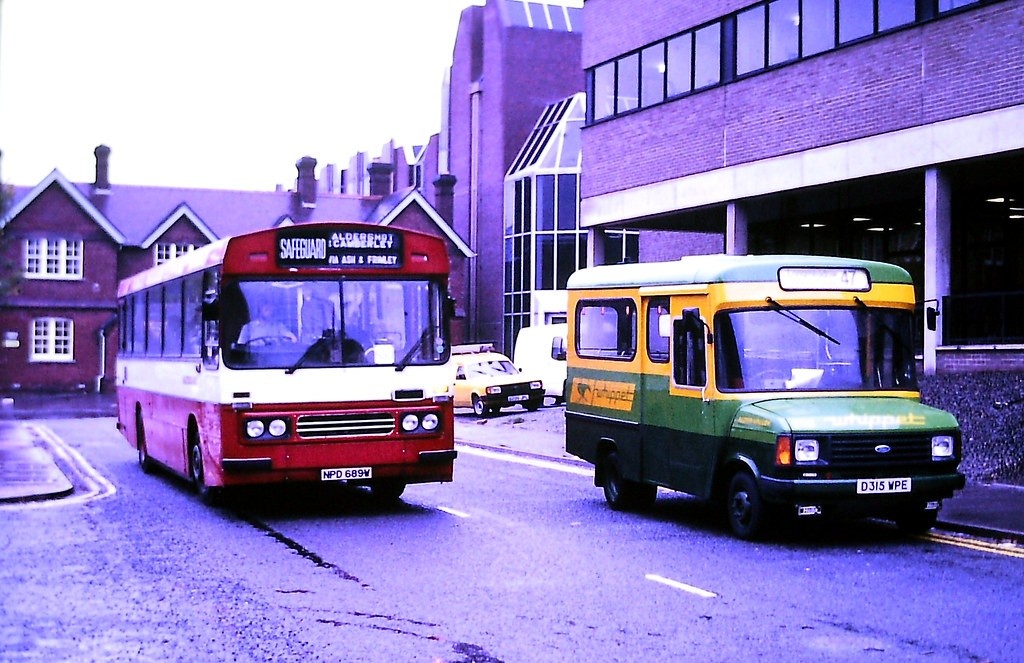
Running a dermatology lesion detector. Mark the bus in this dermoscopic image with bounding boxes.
[113,220,467,507]
[565,250,968,544]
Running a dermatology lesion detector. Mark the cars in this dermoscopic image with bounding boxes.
[450,350,547,417]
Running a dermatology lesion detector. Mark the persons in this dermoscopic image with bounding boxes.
[237,298,298,345]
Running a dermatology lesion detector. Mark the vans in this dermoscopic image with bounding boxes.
[513,322,568,405]
